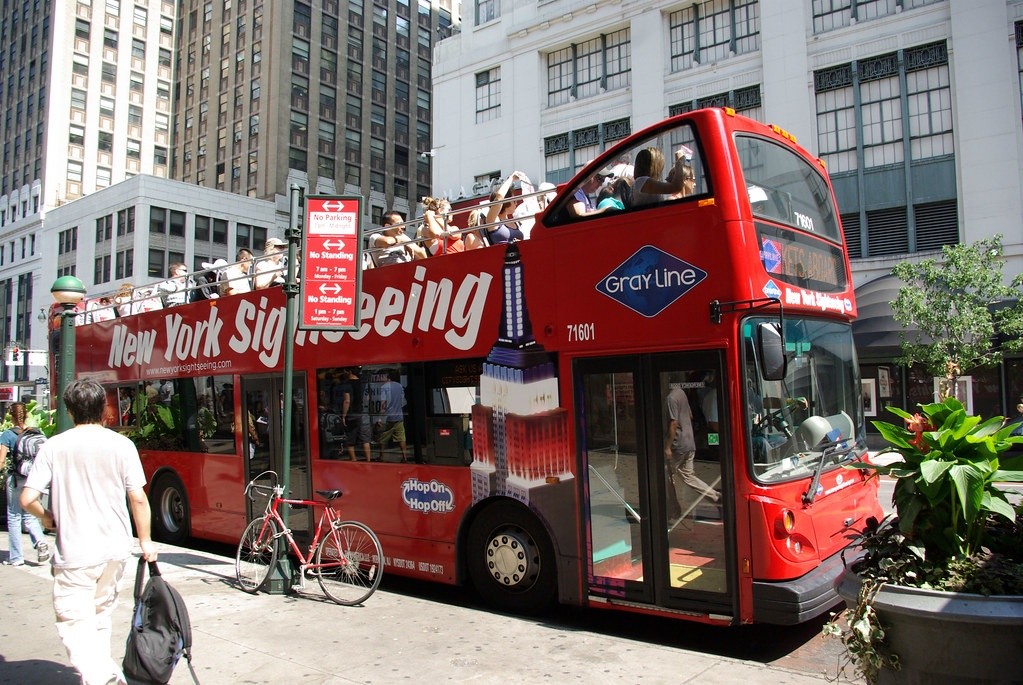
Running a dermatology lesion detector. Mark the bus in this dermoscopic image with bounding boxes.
[46,106,888,630]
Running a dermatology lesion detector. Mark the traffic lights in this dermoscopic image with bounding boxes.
[13,347,18,361]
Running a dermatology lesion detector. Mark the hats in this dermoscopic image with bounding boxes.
[202,259,228,275]
[344,366,362,375]
[264,238,288,250]
[583,158,614,177]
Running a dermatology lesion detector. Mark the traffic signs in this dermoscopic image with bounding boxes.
[301,193,361,332]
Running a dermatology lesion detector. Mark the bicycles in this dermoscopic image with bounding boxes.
[236,470,385,606]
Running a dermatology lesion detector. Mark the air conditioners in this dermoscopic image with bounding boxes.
[236,134,242,141]
[252,127,258,134]
[196,150,203,156]
[185,155,191,161]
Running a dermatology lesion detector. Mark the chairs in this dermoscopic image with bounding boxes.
[247,264,253,290]
[216,270,223,294]
[91,302,98,323]
[420,221,433,257]
[477,210,492,247]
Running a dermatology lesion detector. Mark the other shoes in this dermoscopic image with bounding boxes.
[716,493,723,520]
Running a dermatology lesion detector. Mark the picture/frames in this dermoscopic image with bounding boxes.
[855,379,877,416]
[934,376,974,416]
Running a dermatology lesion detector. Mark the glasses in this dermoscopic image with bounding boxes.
[274,245,285,249]
[176,268,187,272]
[595,174,605,183]
[684,175,696,182]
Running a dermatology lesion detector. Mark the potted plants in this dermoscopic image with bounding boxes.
[821,396,1023,685]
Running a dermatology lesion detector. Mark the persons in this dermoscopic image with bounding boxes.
[375,372,407,462]
[484,171,529,246]
[75,237,302,329]
[661,165,697,202]
[422,197,464,256]
[363,251,375,271]
[662,374,724,521]
[568,144,636,216]
[701,376,809,463]
[746,183,768,213]
[368,211,420,267]
[414,218,435,258]
[631,147,686,207]
[339,365,372,461]
[613,405,680,562]
[0,402,49,566]
[115,376,234,448]
[19,378,157,685]
[535,182,558,212]
[256,389,301,471]
[231,402,260,478]
[465,206,491,251]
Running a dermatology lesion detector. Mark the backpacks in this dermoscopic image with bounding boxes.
[664,458,682,522]
[8,426,48,478]
[3,559,24,565]
[36,539,52,562]
[121,552,193,685]
[321,411,348,444]
